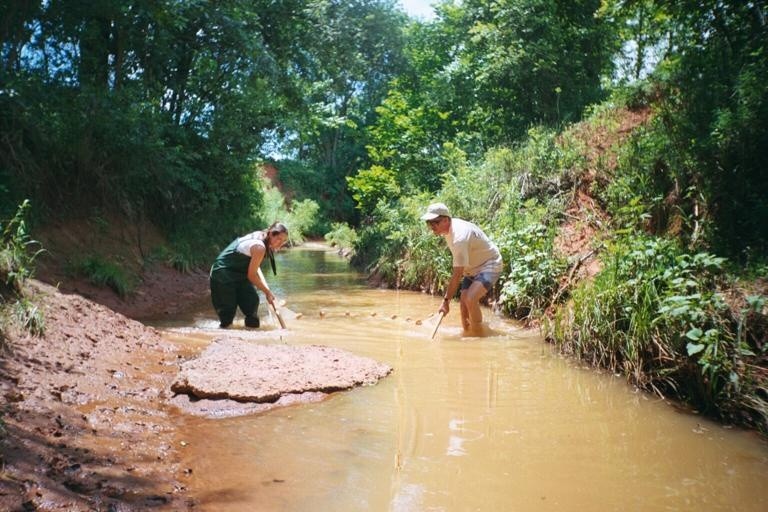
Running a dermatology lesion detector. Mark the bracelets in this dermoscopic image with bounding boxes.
[443,297,449,304]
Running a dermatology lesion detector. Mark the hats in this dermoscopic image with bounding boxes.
[421,203,452,220]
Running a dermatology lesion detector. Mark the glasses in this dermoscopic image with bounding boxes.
[426,219,442,226]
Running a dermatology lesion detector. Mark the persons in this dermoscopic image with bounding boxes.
[209,222,288,329]
[420,203,503,337]
